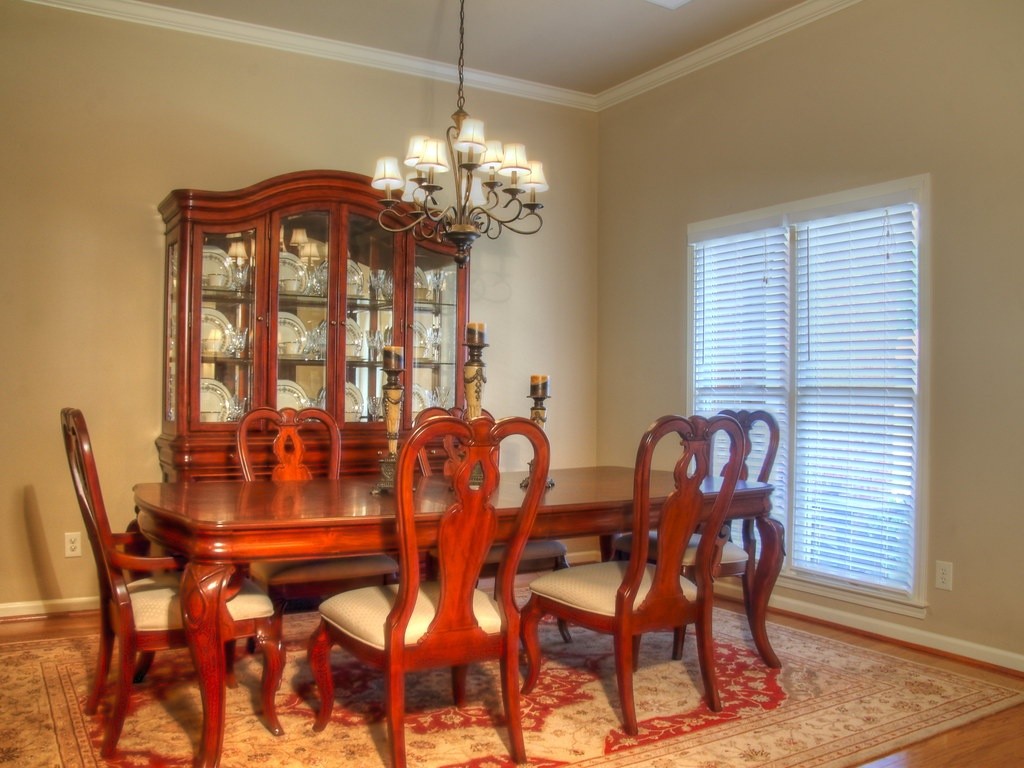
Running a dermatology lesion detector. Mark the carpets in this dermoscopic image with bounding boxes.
[0,606,1024,768]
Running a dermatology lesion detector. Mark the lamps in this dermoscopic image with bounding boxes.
[370,0,549,268]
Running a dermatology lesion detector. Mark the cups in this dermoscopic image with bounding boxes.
[412,345,426,358]
[281,278,300,293]
[413,287,431,300]
[228,396,246,422]
[201,411,221,421]
[202,337,223,355]
[346,281,363,295]
[279,340,299,354]
[204,273,226,287]
[345,344,362,356]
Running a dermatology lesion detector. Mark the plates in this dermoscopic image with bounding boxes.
[411,383,429,425]
[201,306,233,354]
[202,245,235,289]
[385,319,428,347]
[319,258,363,297]
[200,379,233,422]
[278,380,308,412]
[277,311,307,355]
[278,251,309,292]
[318,382,363,422]
[319,317,363,352]
[382,263,429,302]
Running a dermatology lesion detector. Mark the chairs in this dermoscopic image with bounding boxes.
[237,408,399,654]
[518,414,746,737]
[414,406,572,643]
[611,409,779,660]
[307,416,550,768]
[60,407,286,758]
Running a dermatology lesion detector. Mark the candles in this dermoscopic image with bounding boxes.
[531,376,548,396]
[384,345,404,370]
[466,323,484,345]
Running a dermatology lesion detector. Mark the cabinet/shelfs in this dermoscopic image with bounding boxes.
[154,169,471,600]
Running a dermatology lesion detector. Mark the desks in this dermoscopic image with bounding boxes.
[123,466,786,768]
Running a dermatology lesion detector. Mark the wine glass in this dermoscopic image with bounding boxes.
[230,263,250,292]
[436,386,451,410]
[430,271,446,303]
[427,326,440,363]
[227,326,248,358]
[367,396,382,422]
[304,329,319,360]
[366,330,379,361]
[370,269,386,300]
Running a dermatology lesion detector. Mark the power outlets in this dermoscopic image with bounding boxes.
[64,532,81,557]
[935,560,952,590]
